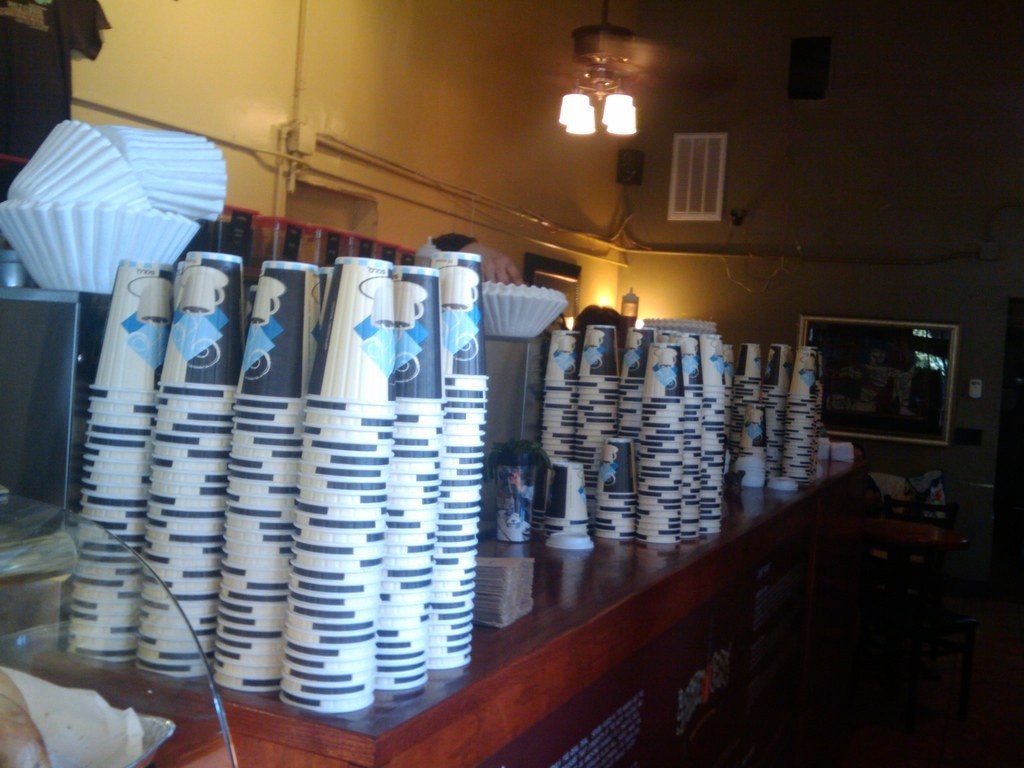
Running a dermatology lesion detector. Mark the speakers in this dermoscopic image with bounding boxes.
[787,37,831,101]
[616,151,645,186]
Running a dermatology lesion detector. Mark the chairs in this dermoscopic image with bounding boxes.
[838,512,979,734]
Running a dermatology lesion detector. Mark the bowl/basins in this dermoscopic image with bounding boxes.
[0,121,227,293]
[483,282,569,338]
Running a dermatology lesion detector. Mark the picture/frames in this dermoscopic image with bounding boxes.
[794,311,961,447]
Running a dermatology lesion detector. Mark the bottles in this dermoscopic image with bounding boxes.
[620,289,638,332]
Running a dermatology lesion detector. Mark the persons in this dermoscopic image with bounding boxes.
[842,441,882,503]
[428,229,628,377]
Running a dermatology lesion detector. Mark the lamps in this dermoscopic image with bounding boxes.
[559,0,638,136]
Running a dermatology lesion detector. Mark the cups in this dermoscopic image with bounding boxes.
[65,249,488,714]
[488,449,540,542]
[531,326,825,549]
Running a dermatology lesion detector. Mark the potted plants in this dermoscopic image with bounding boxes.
[486,438,553,542]
